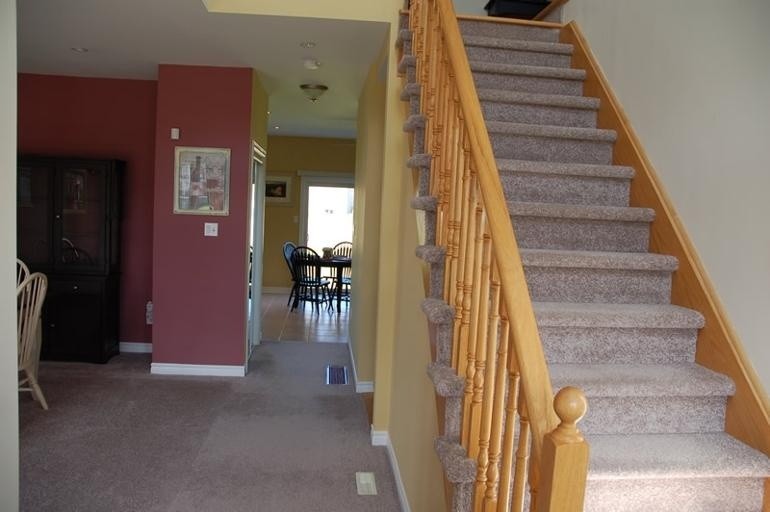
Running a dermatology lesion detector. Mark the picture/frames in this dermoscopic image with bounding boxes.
[265,175,293,203]
[172,146,231,217]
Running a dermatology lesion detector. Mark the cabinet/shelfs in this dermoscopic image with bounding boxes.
[17,152,127,365]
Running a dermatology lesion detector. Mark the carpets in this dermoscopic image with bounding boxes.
[19,340,401,512]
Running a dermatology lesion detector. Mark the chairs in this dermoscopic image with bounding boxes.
[17,258,49,412]
[283,240,353,315]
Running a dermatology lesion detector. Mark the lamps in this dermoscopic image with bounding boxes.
[300,84,328,105]
